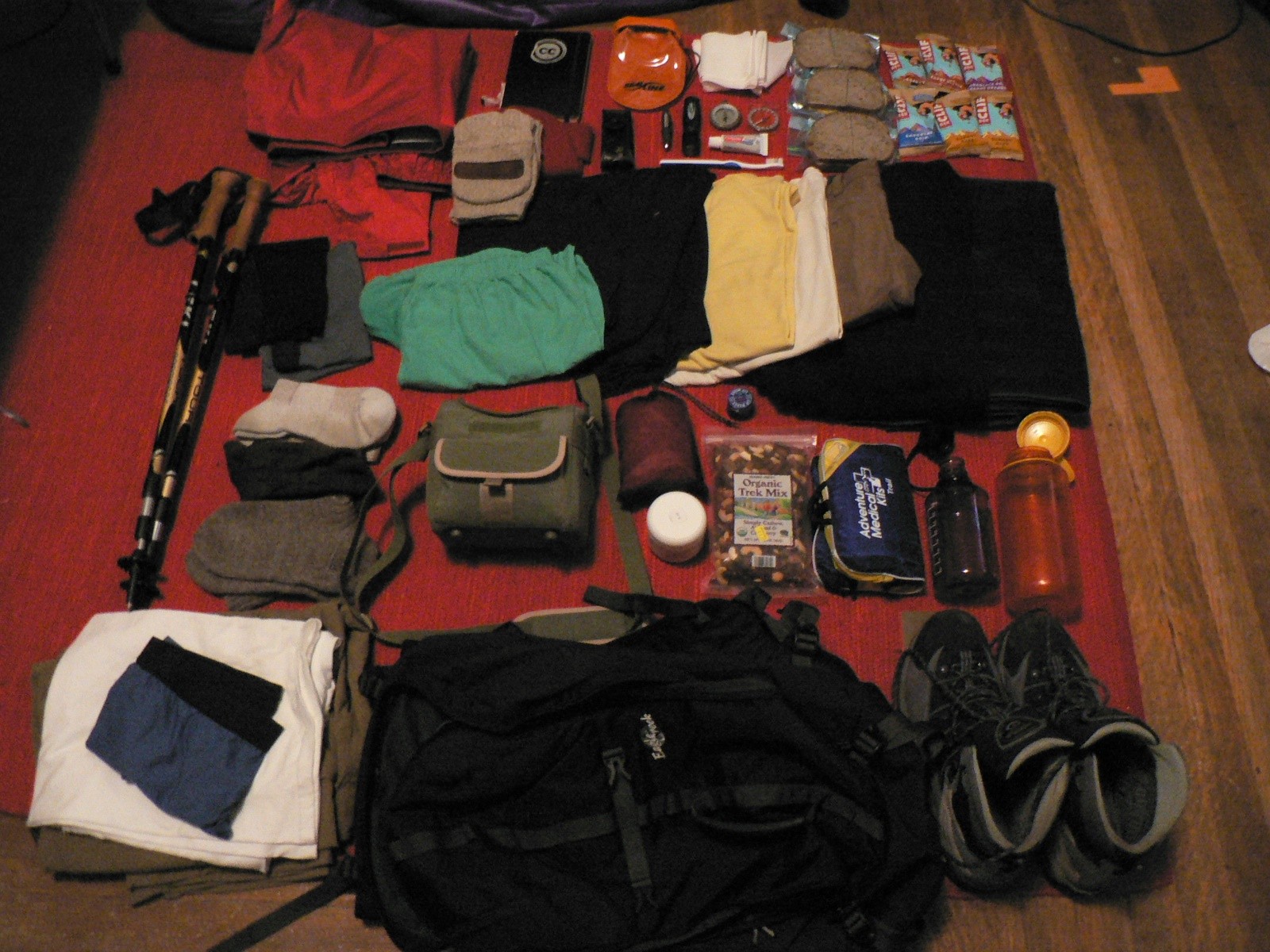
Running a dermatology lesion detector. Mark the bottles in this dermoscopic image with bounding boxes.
[902,421,1000,605]
[994,409,1085,622]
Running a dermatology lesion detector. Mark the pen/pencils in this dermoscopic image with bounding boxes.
[664,111,672,150]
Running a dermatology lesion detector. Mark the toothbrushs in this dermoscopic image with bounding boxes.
[659,157,785,171]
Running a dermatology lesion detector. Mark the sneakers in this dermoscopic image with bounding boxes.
[981,611,1189,906]
[894,604,1079,892]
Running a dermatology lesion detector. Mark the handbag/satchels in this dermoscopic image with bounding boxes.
[811,435,926,596]
[350,582,939,952]
[426,398,598,559]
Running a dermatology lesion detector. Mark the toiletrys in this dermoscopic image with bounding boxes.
[707,133,769,157]
